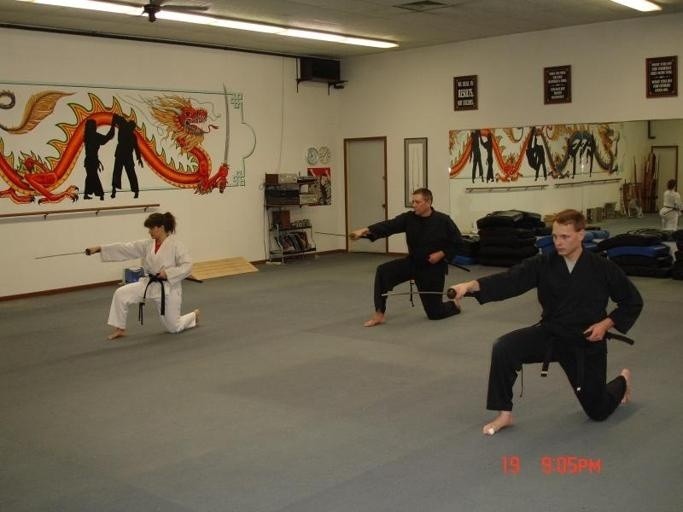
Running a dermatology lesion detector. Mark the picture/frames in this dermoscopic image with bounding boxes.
[453,74,479,112]
[403,136,428,209]
[544,62,573,106]
[645,55,680,98]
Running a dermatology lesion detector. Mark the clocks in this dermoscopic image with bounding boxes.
[319,146,332,164]
[305,147,318,164]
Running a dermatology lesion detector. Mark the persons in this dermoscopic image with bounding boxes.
[448,208,644,435]
[659,179,680,230]
[87,211,200,340]
[351,188,461,326]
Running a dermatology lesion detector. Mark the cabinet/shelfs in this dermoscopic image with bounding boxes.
[266,172,318,208]
[268,224,317,254]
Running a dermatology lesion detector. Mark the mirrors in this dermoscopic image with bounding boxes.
[446,117,683,264]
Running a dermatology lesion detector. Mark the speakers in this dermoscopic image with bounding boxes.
[300,57,340,81]
